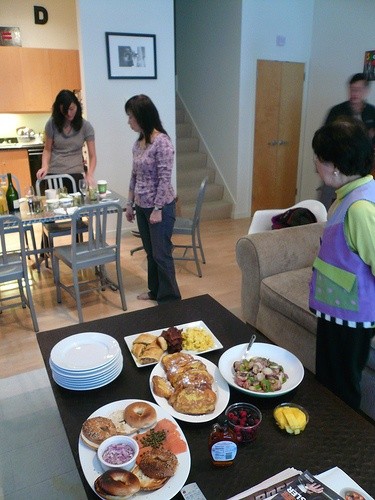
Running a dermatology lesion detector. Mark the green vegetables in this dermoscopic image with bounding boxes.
[135,427,169,451]
[239,359,287,393]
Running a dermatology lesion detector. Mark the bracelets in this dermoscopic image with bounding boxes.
[127,200,133,206]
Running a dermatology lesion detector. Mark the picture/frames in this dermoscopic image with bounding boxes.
[105,32,157,79]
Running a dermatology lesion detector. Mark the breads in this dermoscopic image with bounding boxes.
[81,402,157,450]
[152,353,216,414]
[131,449,178,491]
[95,469,140,500]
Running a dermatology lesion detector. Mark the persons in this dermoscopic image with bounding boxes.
[125,94,181,304]
[273,483,324,500]
[324,72,375,133]
[31,90,96,270]
[308,118,375,411]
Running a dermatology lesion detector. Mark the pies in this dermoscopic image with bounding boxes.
[131,334,165,365]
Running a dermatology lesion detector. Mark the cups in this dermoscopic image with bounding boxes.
[97,180,107,197]
[45,189,73,211]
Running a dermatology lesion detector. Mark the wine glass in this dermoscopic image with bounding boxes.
[78,179,89,206]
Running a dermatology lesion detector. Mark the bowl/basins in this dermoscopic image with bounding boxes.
[224,402,263,442]
[96,435,140,471]
[218,342,306,397]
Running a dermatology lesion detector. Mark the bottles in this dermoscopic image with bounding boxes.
[208,415,238,467]
[6,174,20,215]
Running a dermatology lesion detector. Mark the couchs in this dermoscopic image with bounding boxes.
[236,221,375,420]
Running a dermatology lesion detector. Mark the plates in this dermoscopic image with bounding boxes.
[123,320,223,368]
[78,399,191,500]
[149,354,230,424]
[48,332,124,390]
[274,402,309,430]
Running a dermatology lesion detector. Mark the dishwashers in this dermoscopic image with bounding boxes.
[27,148,87,196]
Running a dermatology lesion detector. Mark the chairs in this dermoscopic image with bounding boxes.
[0,174,207,332]
[247,199,328,233]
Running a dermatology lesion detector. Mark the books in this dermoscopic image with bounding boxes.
[228,466,374,500]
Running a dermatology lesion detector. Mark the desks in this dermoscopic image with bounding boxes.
[0,188,136,256]
[36,294,375,500]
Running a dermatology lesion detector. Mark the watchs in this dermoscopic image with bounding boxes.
[153,207,162,210]
